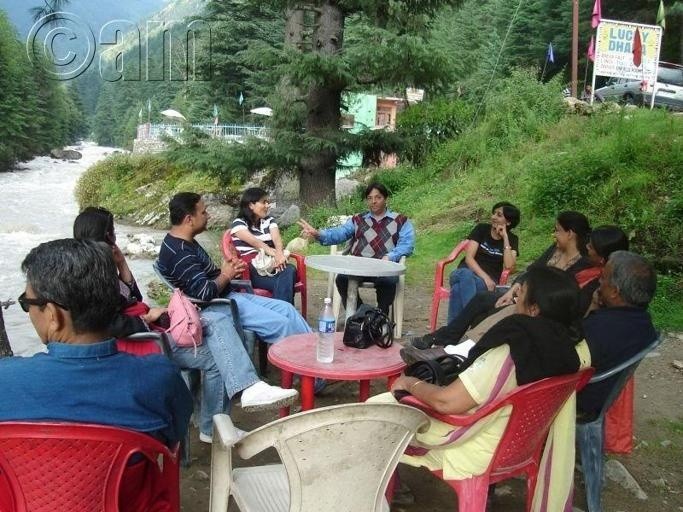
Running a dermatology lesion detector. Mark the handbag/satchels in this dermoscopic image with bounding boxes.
[393,353,465,403]
[249,247,291,277]
[163,287,204,359]
[341,303,397,350]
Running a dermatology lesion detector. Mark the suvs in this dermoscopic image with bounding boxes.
[594,60,683,113]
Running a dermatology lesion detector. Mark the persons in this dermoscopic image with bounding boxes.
[297,183,416,318]
[155,191,348,395]
[1,237,193,467]
[446,201,520,327]
[72,206,297,446]
[229,187,296,306]
[362,266,580,480]
[399,249,660,426]
[410,211,589,350]
[561,224,630,316]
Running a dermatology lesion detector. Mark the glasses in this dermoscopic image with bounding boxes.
[17,292,67,313]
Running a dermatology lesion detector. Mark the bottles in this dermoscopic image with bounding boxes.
[315,297,336,363]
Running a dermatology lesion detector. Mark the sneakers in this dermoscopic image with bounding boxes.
[240,381,300,414]
[399,346,448,365]
[410,332,443,350]
[199,424,249,445]
[318,379,348,397]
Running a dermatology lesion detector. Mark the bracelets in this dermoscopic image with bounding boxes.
[502,246,513,249]
[220,271,230,282]
[408,379,424,393]
